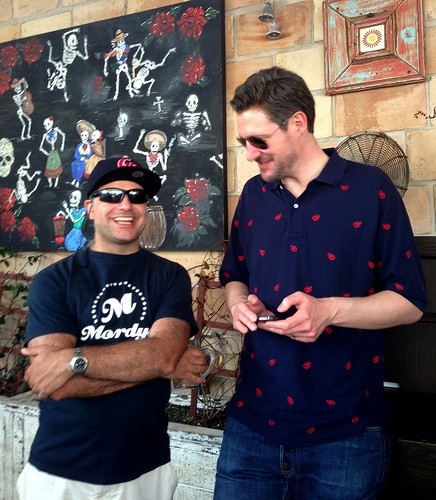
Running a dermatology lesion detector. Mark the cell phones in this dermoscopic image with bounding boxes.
[256,315,280,323]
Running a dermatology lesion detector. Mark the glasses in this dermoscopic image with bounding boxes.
[88,188,149,204]
[236,116,290,149]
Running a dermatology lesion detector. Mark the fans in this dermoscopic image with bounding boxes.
[342,132,409,199]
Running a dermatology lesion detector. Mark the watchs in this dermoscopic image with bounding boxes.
[69,347,89,374]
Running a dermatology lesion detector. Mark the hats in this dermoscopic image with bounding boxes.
[82,155,162,199]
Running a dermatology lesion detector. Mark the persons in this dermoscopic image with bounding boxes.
[16,156,206,500]
[213,65,427,500]
